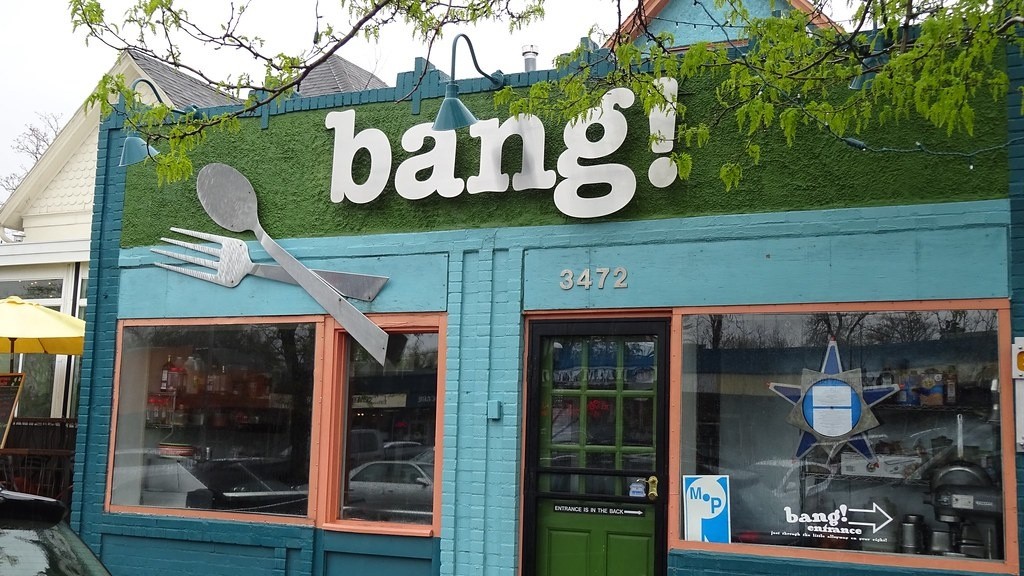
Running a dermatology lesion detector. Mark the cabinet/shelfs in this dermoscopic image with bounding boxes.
[148,392,306,515]
[789,380,1008,565]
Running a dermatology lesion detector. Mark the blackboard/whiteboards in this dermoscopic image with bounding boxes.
[0,372,27,450]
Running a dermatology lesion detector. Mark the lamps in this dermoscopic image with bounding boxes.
[119,78,202,169]
[432,33,506,132]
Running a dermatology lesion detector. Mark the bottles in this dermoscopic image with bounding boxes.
[149,353,271,427]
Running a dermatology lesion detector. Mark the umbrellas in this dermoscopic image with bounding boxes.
[0,294,86,376]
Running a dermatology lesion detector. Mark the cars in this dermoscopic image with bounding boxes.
[0,487,111,576]
[546,433,943,520]
[109,427,436,530]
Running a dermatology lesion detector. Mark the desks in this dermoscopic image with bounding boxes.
[0,448,73,501]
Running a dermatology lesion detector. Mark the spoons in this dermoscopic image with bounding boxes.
[195,163,391,366]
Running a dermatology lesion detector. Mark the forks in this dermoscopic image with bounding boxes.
[150,224,391,305]
